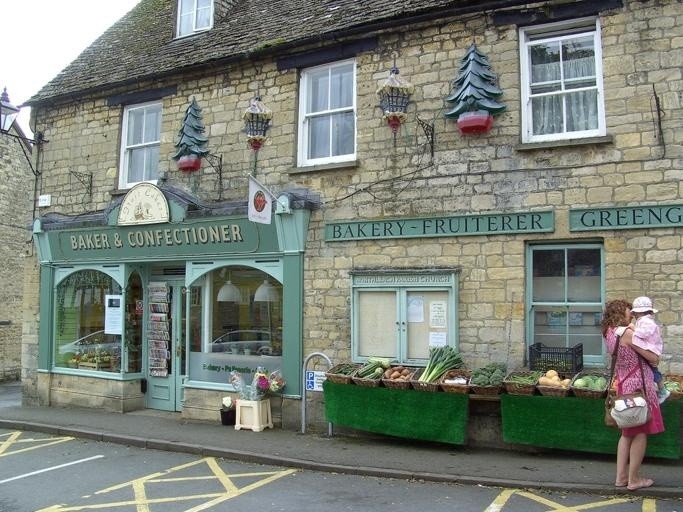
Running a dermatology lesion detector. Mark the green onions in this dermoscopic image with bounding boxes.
[418,344,464,383]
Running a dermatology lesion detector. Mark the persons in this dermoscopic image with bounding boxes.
[628,297,671,406]
[598,297,665,492]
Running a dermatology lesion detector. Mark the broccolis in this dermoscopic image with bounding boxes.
[472,361,507,388]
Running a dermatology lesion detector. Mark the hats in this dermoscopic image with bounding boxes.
[629,296,659,314]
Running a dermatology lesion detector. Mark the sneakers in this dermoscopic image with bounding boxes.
[657,387,670,404]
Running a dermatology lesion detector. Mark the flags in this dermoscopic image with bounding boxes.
[245,177,274,228]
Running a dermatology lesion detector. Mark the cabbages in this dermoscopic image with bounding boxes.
[574,370,607,391]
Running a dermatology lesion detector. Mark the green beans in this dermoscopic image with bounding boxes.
[508,368,545,385]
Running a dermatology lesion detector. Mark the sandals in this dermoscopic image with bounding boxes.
[626,476,654,490]
[614,475,629,486]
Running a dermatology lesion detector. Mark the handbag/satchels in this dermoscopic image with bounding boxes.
[604,391,653,429]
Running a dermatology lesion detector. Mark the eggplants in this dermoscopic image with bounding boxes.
[357,355,391,380]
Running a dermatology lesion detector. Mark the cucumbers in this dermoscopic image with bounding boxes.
[529,356,571,372]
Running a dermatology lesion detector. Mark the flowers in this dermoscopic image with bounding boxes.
[221,397,234,412]
[229,365,286,401]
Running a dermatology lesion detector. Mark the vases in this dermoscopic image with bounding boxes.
[220,409,236,426]
[233,399,274,432]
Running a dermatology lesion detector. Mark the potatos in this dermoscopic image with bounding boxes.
[384,366,411,381]
[539,370,570,388]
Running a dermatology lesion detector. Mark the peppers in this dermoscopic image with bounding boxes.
[333,363,356,376]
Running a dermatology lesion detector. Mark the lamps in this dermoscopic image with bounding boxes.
[376,54,414,134]
[241,82,272,149]
[216,265,280,302]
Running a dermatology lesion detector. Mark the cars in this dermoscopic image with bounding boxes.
[53,326,140,359]
[205,328,278,357]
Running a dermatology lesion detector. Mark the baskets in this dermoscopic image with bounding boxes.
[325,362,469,394]
[468,367,683,400]
